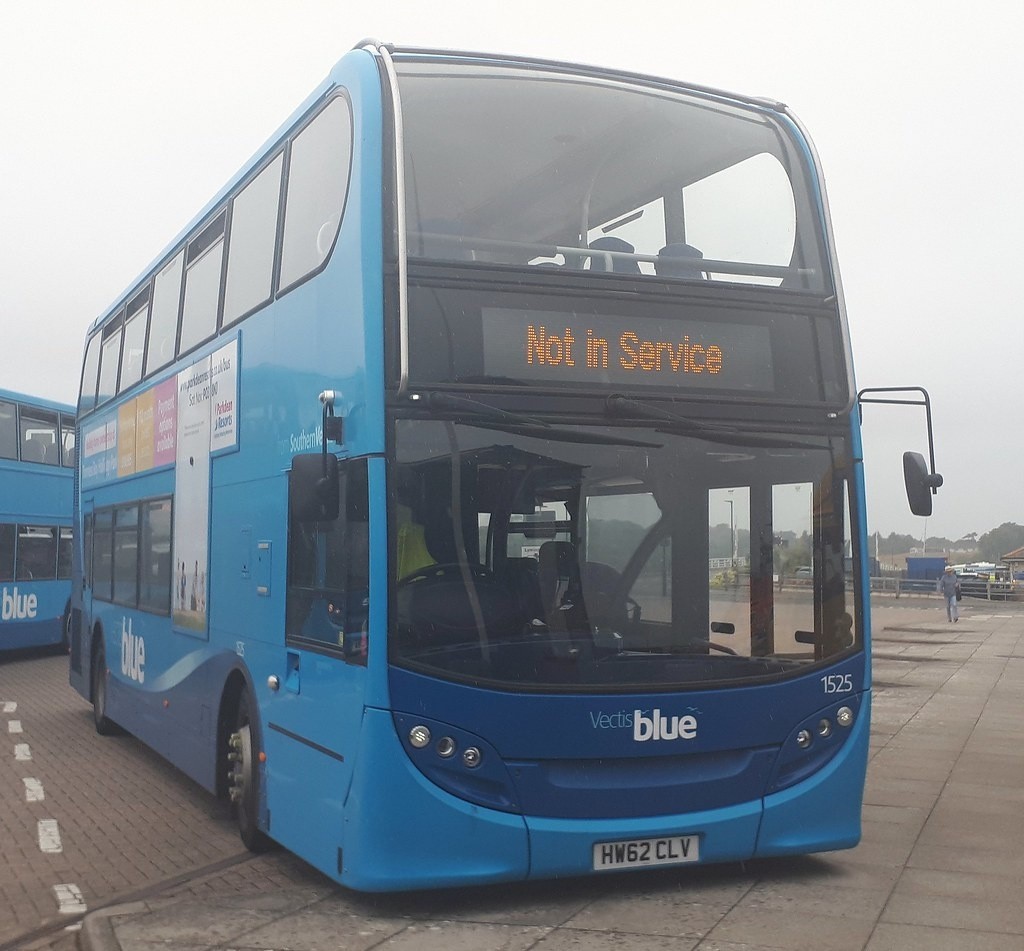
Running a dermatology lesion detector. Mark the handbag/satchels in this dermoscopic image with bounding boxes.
[956,578,961,601]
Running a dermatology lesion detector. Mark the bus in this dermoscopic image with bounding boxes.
[0,387,81,657]
[69,30,946,897]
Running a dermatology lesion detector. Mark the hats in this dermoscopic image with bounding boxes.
[945,566,953,572]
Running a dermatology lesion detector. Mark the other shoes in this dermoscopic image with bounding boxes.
[954,618,958,623]
[948,619,952,622]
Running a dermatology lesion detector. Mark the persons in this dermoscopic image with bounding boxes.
[778,570,784,591]
[939,565,960,622]
[722,567,729,591]
[397,476,462,586]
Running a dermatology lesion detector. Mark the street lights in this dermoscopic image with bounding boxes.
[723,499,735,568]
